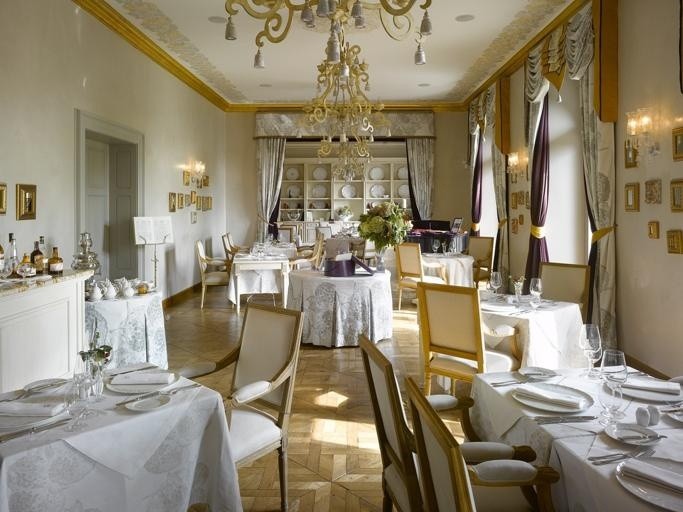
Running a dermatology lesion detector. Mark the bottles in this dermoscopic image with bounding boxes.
[18,253,36,277]
[89,326,103,396]
[636,407,650,427]
[0,233,18,278]
[31,241,43,274]
[49,246,64,276]
[39,236,49,271]
[10,240,20,275]
[646,406,660,427]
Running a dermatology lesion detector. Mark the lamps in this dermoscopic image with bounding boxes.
[224,0,434,180]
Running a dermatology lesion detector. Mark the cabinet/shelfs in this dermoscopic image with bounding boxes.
[0,268,97,392]
[276,156,412,243]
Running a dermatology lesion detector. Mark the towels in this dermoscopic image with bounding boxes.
[621,377,681,395]
[0,399,68,417]
[621,457,683,494]
[110,371,175,385]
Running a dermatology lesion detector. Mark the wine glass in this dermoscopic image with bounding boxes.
[579,324,601,380]
[583,339,603,376]
[491,271,503,302]
[64,388,88,429]
[281,211,287,222]
[599,380,623,428]
[529,277,543,298]
[430,239,441,258]
[75,349,99,417]
[600,350,627,417]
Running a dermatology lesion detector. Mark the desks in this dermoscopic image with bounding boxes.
[85,283,169,372]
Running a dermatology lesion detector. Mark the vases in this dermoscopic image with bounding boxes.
[87,362,103,398]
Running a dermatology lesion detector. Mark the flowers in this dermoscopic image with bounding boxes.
[77,331,112,370]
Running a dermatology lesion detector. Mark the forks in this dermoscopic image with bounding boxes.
[592,449,656,466]
[588,445,641,461]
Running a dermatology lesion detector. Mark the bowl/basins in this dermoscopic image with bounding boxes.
[288,211,302,221]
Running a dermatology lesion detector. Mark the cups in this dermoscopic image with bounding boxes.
[134,278,140,285]
[147,282,153,289]
[399,199,407,210]
[141,280,147,285]
[383,195,390,198]
[136,284,147,296]
[23,268,36,287]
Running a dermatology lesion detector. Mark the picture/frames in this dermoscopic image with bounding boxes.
[624,126,683,254]
[168,170,212,224]
[16,184,37,221]
[510,164,529,234]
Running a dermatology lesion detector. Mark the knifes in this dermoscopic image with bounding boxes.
[537,419,589,424]
[111,365,158,378]
[524,372,562,376]
[616,435,667,439]
[116,390,161,406]
[0,418,72,443]
[534,415,599,421]
[0,422,68,440]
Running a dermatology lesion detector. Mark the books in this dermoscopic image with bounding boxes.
[3,393,65,416]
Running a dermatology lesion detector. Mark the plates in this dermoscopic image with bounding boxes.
[313,168,327,179]
[0,397,66,428]
[134,294,151,297]
[606,424,663,446]
[371,185,385,198]
[107,371,181,392]
[518,367,556,378]
[371,200,387,208]
[286,167,299,180]
[398,184,410,197]
[124,394,172,411]
[23,379,66,394]
[370,167,385,179]
[311,184,328,198]
[607,381,683,402]
[667,403,683,422]
[616,456,683,512]
[342,184,356,198]
[511,383,595,413]
[286,185,301,197]
[397,166,410,180]
[146,286,161,293]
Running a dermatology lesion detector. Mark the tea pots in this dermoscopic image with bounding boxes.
[89,276,134,300]
